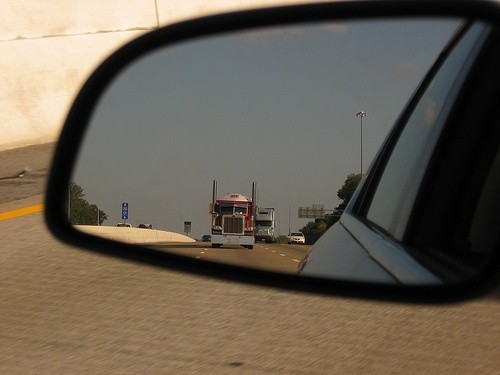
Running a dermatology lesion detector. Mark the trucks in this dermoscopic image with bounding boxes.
[254,206,276,242]
[138,223,152,229]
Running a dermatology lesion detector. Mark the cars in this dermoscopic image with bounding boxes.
[286,232,306,244]
[202,234,211,241]
[115,223,132,228]
[296,11,496,284]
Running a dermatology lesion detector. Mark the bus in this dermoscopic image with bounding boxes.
[209,178,256,250]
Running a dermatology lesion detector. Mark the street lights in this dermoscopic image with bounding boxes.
[287,200,291,235]
[356,111,369,177]
